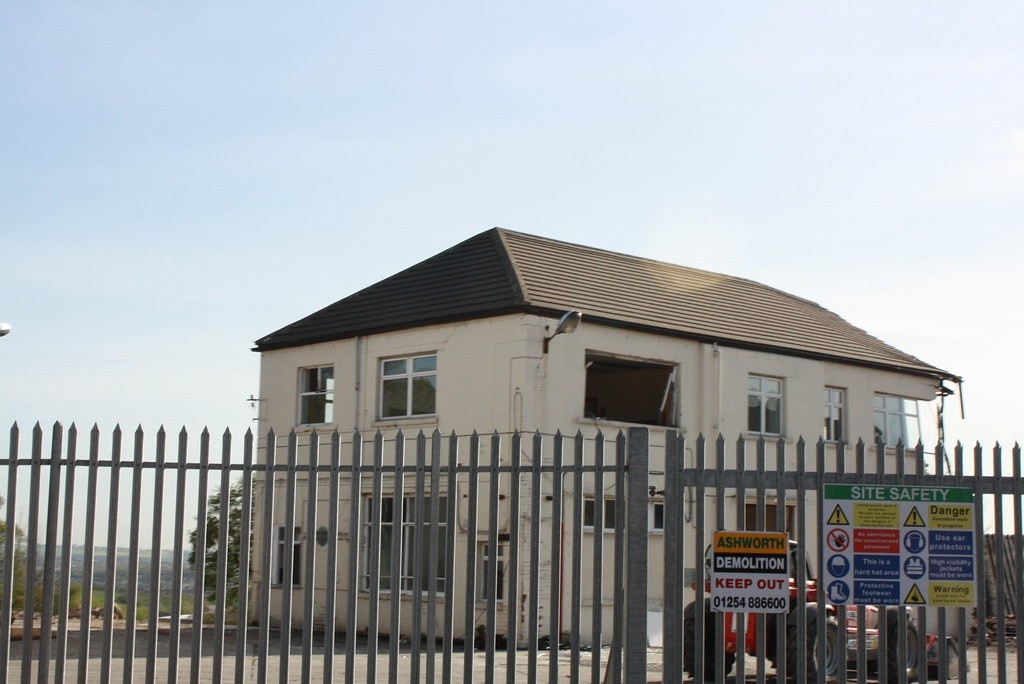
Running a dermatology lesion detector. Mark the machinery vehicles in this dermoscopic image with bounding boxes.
[683,536,970,684]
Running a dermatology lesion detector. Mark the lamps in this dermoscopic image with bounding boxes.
[543,308,582,354]
